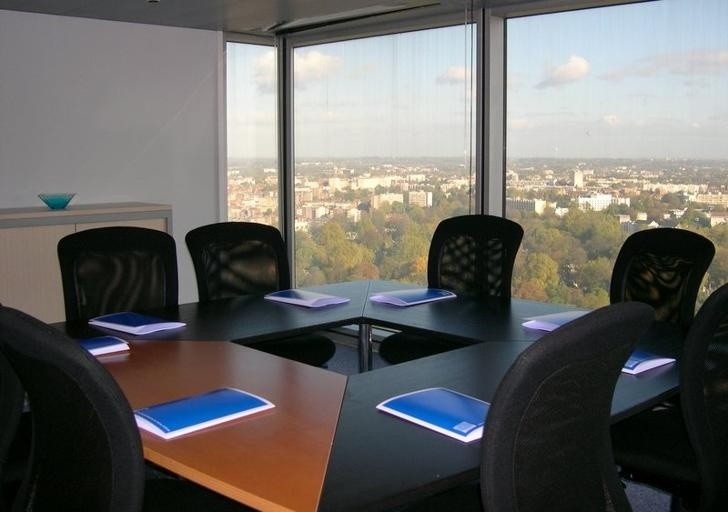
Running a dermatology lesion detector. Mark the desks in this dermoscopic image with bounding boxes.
[32,279,686,511]
[0,201,172,323]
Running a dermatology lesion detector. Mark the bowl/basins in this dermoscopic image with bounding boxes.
[40,192,75,209]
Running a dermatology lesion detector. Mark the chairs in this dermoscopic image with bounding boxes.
[609,228,714,336]
[479,298,653,512]
[618,284,727,511]
[57,225,179,331]
[187,222,336,366]
[380,215,523,362]
[0,306,145,511]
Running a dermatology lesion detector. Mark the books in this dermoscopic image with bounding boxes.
[369,289,458,308]
[376,388,491,444]
[78,337,131,357]
[265,288,351,308]
[621,348,680,375]
[89,312,188,335]
[520,309,594,332]
[135,386,277,441]
